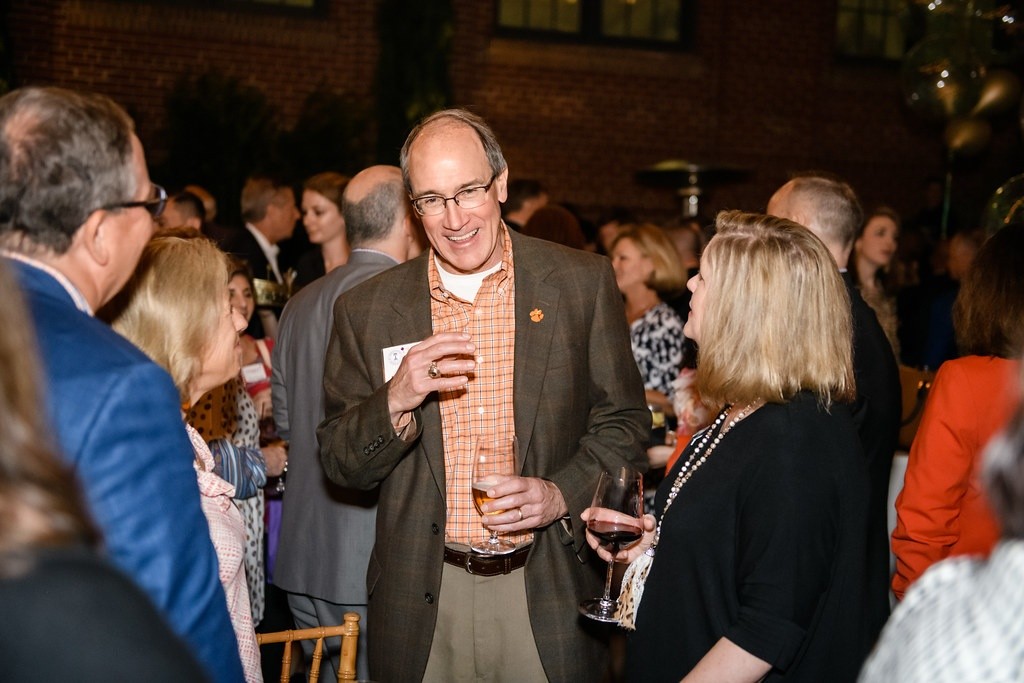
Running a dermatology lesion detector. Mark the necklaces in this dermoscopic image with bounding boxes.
[611,394,760,629]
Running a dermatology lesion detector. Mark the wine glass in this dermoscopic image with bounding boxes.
[467,435,520,556]
[577,465,644,624]
[255,400,288,497]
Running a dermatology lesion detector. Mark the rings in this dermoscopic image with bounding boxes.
[285,462,288,465]
[516,508,524,521]
[284,467,288,472]
[428,362,441,379]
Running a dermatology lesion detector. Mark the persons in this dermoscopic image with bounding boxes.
[612,225,689,516]
[94,235,264,683]
[0,255,213,682]
[315,108,655,683]
[0,85,247,683]
[858,400,1024,682]
[154,176,986,367]
[187,366,289,630]
[579,209,893,683]
[888,225,1024,602]
[269,165,431,683]
[223,259,278,451]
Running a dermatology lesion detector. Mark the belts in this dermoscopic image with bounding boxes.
[444,541,533,577]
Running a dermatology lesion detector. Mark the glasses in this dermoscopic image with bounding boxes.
[87,183,167,222]
[408,173,500,217]
